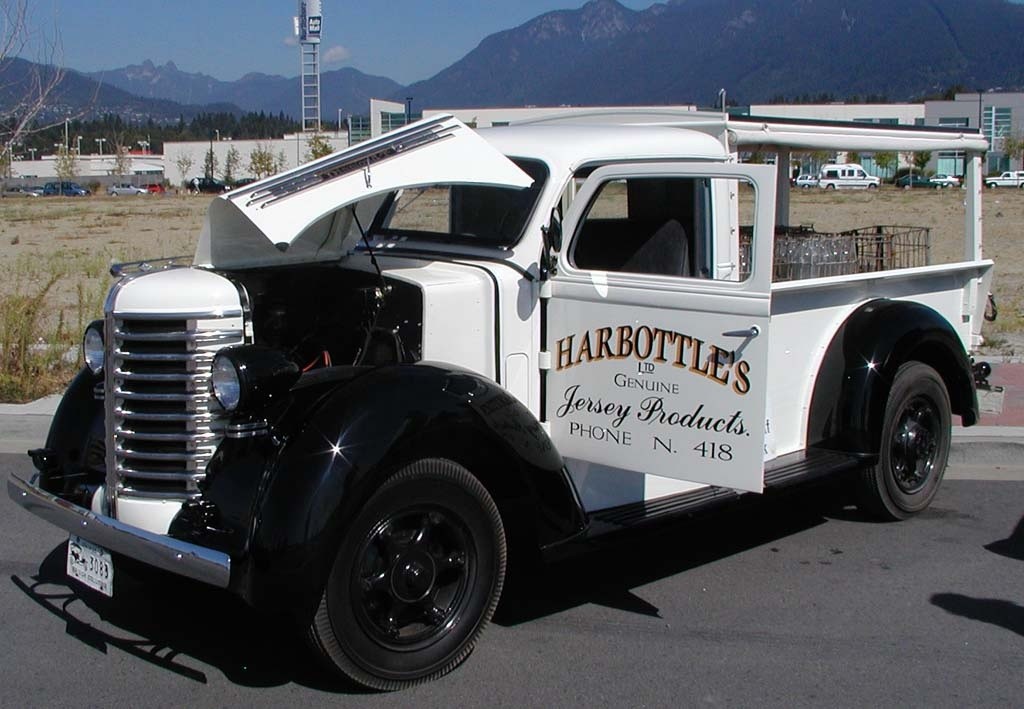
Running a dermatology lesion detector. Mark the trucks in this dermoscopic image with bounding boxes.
[2,109,995,697]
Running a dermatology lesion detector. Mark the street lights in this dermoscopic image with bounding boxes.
[137,140,150,154]
[94,137,106,155]
[75,136,83,155]
[28,147,37,160]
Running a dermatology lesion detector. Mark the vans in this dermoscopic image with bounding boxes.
[814,162,881,192]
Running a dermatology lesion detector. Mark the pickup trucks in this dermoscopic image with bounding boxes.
[983,170,1024,190]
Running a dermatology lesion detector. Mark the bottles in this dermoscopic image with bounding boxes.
[739,231,855,283]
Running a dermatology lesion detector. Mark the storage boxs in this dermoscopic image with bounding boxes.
[837,222,932,274]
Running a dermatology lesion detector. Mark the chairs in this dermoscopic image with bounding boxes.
[618,205,693,274]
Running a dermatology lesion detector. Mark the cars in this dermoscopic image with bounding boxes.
[795,174,819,191]
[44,181,90,197]
[185,177,231,193]
[927,173,960,187]
[106,182,150,197]
[29,185,43,194]
[230,177,255,189]
[142,181,166,194]
[893,169,938,190]
[5,181,38,197]
